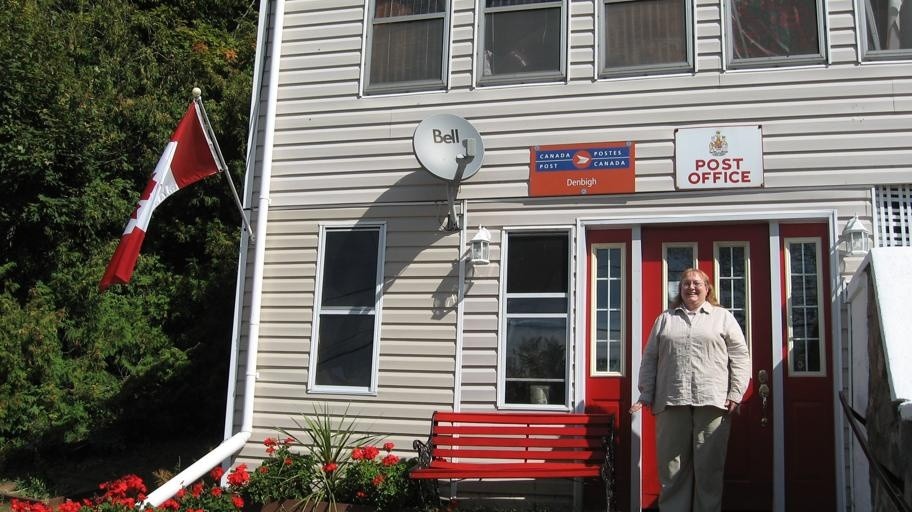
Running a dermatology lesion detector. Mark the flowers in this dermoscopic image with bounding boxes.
[93,473,146,509]
[156,464,249,512]
[11,498,92,511]
[261,437,401,499]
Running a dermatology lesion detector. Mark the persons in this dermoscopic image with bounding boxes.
[628,268,750,512]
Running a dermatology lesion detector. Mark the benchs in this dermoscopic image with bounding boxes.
[408,411,616,512]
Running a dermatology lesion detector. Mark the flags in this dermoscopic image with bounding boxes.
[98,100,223,291]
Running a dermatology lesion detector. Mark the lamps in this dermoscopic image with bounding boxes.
[469,227,491,265]
[843,218,868,255]
